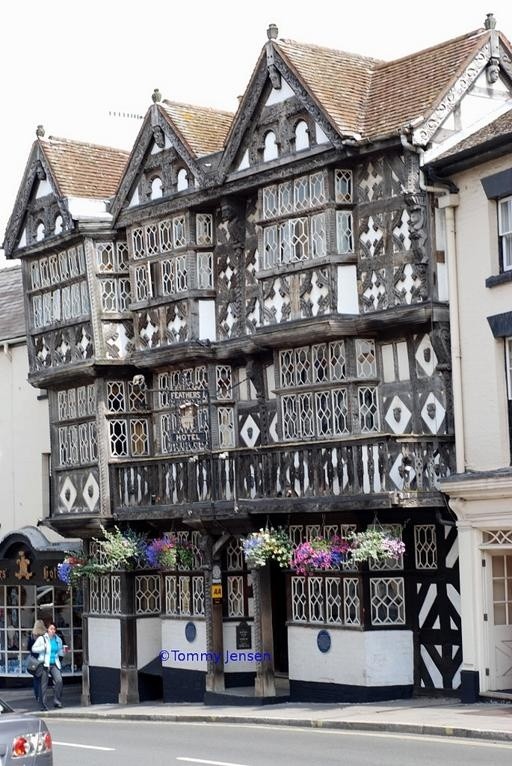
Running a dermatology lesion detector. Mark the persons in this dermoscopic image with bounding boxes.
[2,616,20,665]
[31,622,68,710]
[27,620,48,704]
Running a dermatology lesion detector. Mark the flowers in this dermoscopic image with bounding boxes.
[240,525,408,574]
[51,524,191,591]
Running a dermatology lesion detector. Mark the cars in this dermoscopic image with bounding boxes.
[0,697,53,766]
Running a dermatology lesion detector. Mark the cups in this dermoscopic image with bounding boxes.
[63,644,69,654]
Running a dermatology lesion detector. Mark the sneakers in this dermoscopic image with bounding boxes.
[38,701,63,712]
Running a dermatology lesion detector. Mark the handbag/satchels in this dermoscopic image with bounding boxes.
[26,655,44,679]
